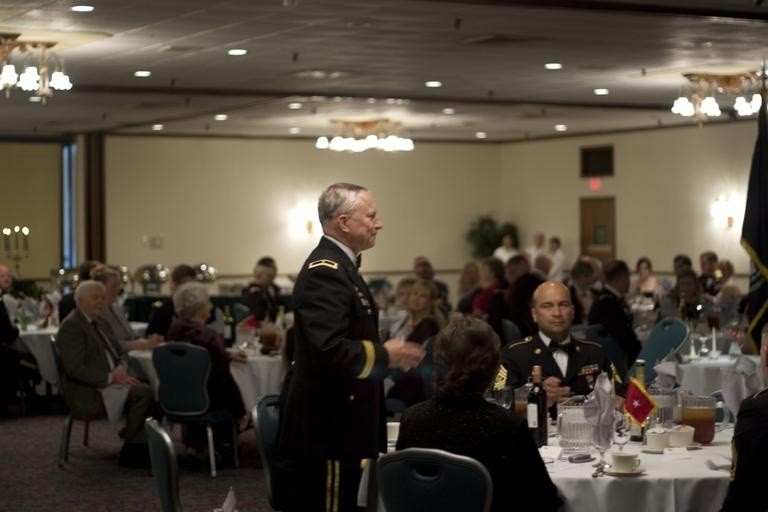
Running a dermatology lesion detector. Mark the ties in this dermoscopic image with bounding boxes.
[91,320,122,368]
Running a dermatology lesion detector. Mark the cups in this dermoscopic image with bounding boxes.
[556,400,589,458]
[668,427,694,446]
[514,387,528,422]
[681,394,730,447]
[610,452,641,473]
[643,430,669,450]
[644,387,679,431]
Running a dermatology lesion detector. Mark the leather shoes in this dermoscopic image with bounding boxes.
[120,442,152,477]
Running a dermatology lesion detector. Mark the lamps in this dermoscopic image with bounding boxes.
[0,32,73,107]
[315,116,416,155]
[670,71,766,122]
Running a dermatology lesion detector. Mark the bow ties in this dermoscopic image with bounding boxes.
[355,252,362,270]
[548,339,573,356]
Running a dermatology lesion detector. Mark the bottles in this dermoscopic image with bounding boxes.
[223,305,234,346]
[525,364,547,449]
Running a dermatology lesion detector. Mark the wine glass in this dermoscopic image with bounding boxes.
[610,415,632,453]
[590,422,612,470]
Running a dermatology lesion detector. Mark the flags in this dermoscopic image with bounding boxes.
[622,376,656,428]
[736,89,767,357]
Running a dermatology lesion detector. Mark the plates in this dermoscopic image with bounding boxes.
[641,446,673,454]
[603,467,646,476]
[666,442,701,451]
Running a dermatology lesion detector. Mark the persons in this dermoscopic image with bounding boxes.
[669,256,691,297]
[718,322,768,511]
[585,259,642,376]
[412,257,449,314]
[241,258,280,318]
[364,278,389,343]
[88,265,165,351]
[699,252,719,296]
[396,316,567,512]
[565,255,603,323]
[0,299,41,411]
[0,265,26,300]
[486,280,530,315]
[392,280,448,367]
[637,257,661,297]
[674,269,710,325]
[455,279,485,316]
[699,260,741,324]
[165,282,248,467]
[455,231,565,280]
[143,265,215,336]
[272,182,428,512]
[486,280,626,418]
[56,280,154,466]
[389,277,419,329]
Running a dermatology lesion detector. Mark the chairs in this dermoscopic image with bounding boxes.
[623,318,691,385]
[50,334,122,466]
[250,394,281,509]
[151,341,239,476]
[373,447,495,511]
[143,417,188,512]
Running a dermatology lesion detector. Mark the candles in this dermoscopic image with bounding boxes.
[6,229,10,249]
[13,226,20,248]
[22,227,28,249]
[710,326,716,351]
[3,228,7,248]
